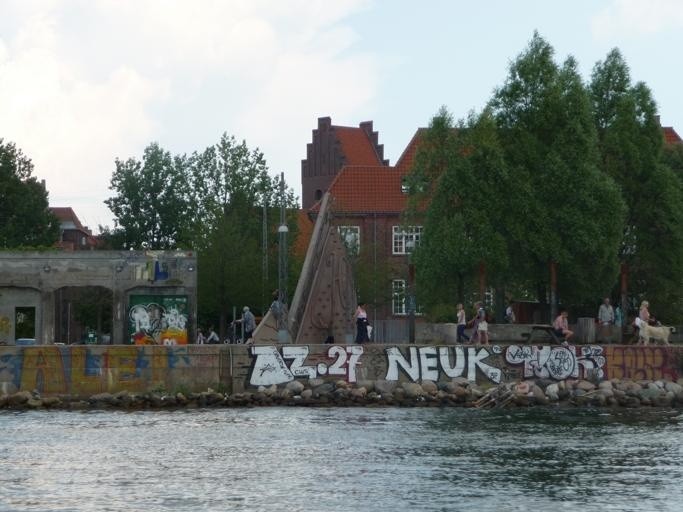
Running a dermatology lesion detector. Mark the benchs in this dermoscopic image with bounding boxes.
[521,333,531,345]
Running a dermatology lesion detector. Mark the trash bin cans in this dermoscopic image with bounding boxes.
[577,317,596,345]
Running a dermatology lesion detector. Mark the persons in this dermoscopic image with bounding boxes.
[466,303,479,345]
[197,328,206,344]
[474,301,489,345]
[351,302,370,344]
[456,303,469,344]
[231,306,257,344]
[270,297,288,329]
[205,327,220,344]
[505,300,516,323]
[599,297,660,345]
[552,307,574,346]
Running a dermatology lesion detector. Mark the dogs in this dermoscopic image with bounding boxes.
[638,320,676,347]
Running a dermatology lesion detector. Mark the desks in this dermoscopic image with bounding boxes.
[528,325,561,346]
